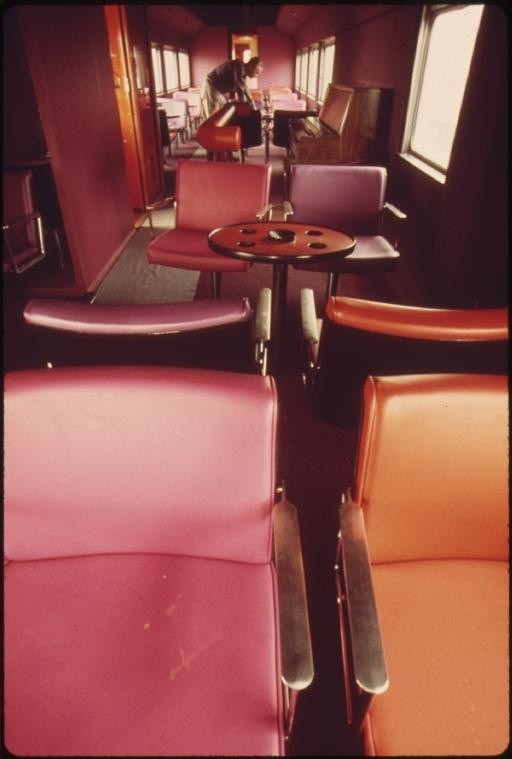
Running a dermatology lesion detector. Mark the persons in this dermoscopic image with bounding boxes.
[197,55,264,163]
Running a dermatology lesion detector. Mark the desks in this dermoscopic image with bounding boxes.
[208,221,357,350]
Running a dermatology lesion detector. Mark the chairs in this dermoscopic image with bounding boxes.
[3,364,316,759]
[146,159,273,297]
[282,162,408,297]
[335,373,510,759]
[299,288,508,429]
[152,79,384,161]
[24,285,271,377]
